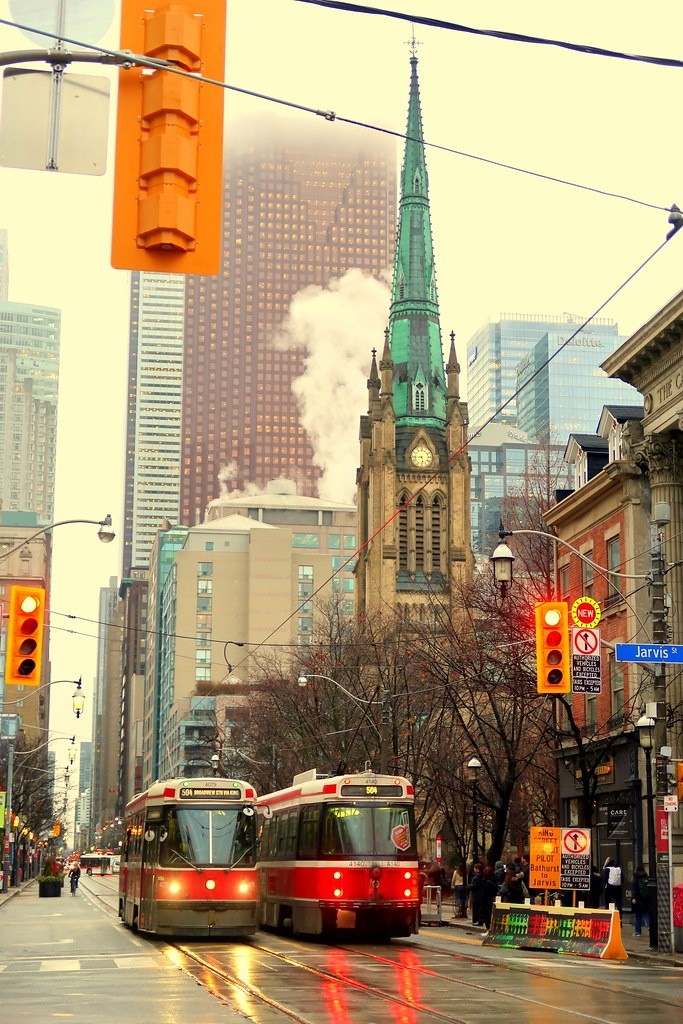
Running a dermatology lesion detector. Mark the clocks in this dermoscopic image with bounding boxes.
[410,445,433,468]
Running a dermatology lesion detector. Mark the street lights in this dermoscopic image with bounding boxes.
[491,521,672,955]
[297,670,391,775]
[211,746,285,791]
[2,734,77,893]
[10,766,71,888]
[634,711,659,949]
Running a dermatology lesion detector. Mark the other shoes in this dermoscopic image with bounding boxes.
[455,913,468,918]
[631,932,642,937]
[473,922,483,926]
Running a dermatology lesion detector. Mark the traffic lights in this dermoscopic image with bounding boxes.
[535,602,570,693]
[3,584,46,687]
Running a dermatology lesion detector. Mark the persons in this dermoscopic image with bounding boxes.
[451,864,462,912]
[602,857,623,927]
[630,864,649,937]
[495,856,537,904]
[68,862,81,893]
[480,866,497,936]
[469,863,484,927]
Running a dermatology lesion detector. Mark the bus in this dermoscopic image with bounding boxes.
[79,853,122,877]
[119,776,259,936]
[260,767,422,941]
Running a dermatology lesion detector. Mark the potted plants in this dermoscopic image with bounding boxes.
[36,875,61,897]
